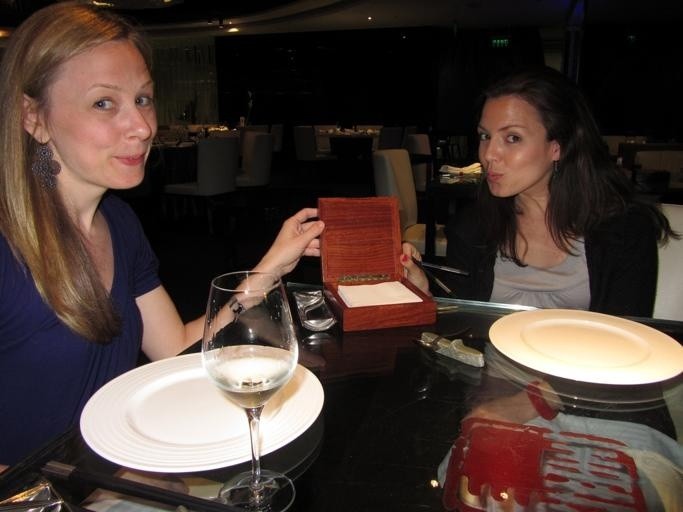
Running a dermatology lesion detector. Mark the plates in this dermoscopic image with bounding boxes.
[482,350,679,412]
[79,347,324,474]
[489,309,683,385]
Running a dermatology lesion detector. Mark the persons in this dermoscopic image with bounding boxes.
[0,1,327,479]
[398,67,682,319]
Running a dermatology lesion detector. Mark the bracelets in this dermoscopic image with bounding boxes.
[225,295,247,316]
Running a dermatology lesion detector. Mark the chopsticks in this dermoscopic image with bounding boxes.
[36,456,248,512]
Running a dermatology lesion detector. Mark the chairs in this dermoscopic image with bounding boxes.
[168,122,455,256]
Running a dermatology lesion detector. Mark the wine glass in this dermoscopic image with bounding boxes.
[200,269,299,510]
